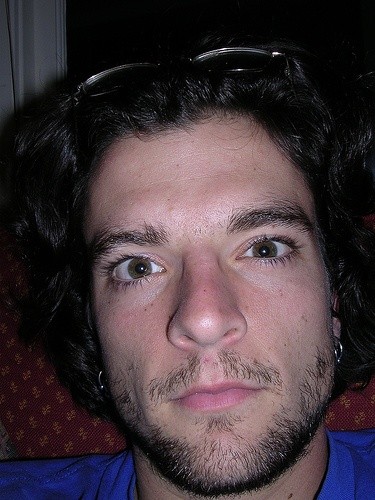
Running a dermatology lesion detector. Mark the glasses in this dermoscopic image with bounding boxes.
[69,46,305,109]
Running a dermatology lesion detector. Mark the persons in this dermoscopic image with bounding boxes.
[0,36,373,500]
